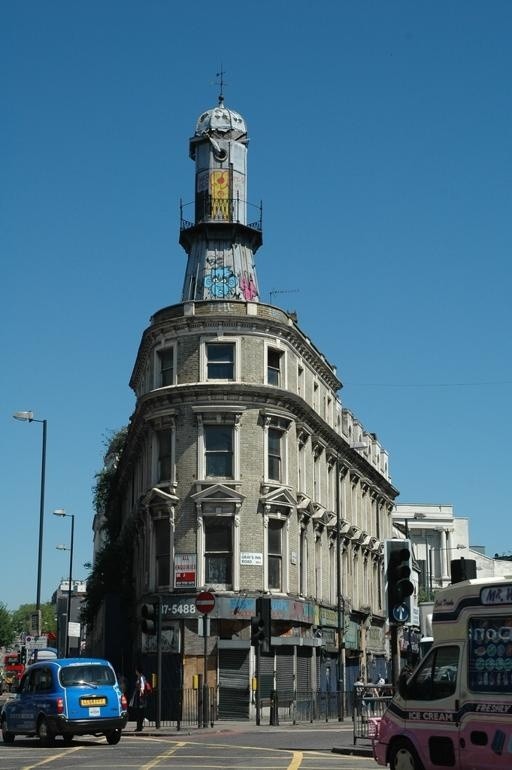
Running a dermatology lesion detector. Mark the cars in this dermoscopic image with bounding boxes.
[1,657,130,745]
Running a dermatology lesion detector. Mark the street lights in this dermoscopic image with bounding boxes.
[11,410,48,664]
[428,544,467,597]
[54,508,74,657]
[335,441,370,723]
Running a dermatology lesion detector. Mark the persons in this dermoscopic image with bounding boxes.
[128,666,161,732]
[377,678,394,707]
[350,676,364,721]
[362,677,380,723]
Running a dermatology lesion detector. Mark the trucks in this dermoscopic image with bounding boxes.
[374,573,512,770]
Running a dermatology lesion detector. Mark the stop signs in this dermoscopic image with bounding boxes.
[195,592,216,615]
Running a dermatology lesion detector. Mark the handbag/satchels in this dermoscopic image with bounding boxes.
[137,683,153,697]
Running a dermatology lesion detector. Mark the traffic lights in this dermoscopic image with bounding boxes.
[142,602,158,636]
[21,650,27,664]
[250,615,264,647]
[387,540,414,624]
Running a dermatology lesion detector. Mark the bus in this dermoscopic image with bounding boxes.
[3,652,25,680]
